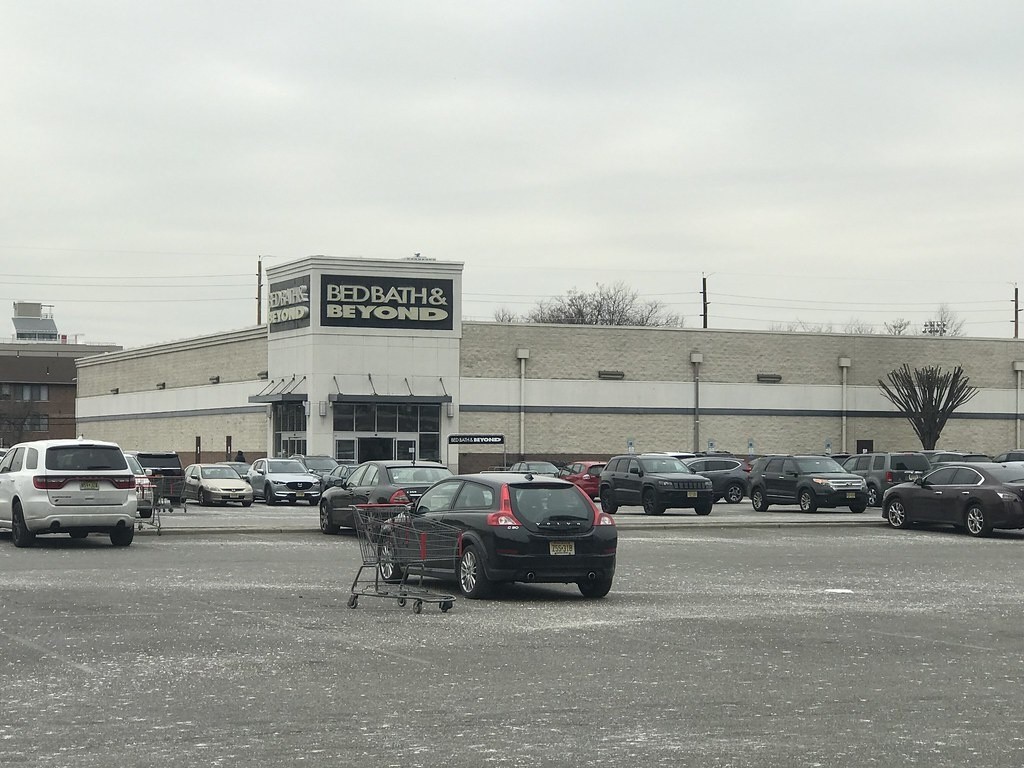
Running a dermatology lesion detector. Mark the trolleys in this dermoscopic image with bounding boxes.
[133,474,162,537]
[156,475,188,514]
[347,505,462,615]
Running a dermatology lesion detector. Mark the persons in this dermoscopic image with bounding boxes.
[234,451,245,462]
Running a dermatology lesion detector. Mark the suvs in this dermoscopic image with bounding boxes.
[553,460,608,502]
[671,457,751,505]
[748,454,870,514]
[246,458,321,506]
[0,435,138,549]
[694,450,1024,469]
[840,451,933,507]
[122,450,186,507]
[598,452,714,517]
[289,454,339,479]
[508,461,560,478]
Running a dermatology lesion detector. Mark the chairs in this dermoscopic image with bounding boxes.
[461,490,573,521]
[413,471,428,482]
[47,453,92,469]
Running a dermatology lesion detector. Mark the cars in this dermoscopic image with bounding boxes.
[318,464,363,499]
[123,453,155,518]
[377,470,618,600]
[205,462,251,480]
[881,462,1024,538]
[180,463,253,507]
[320,460,466,543]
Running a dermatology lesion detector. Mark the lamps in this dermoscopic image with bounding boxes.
[447,402,454,417]
[111,388,118,394]
[319,401,327,416]
[209,376,219,383]
[599,371,625,379]
[156,382,165,389]
[757,373,782,383]
[257,371,268,379]
